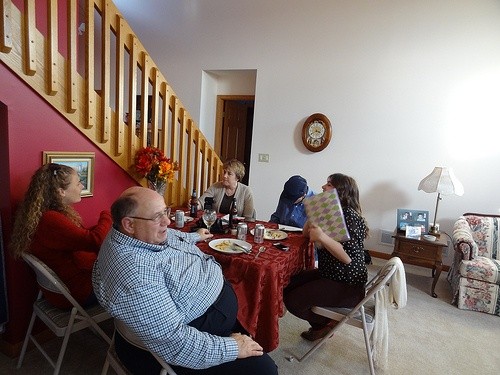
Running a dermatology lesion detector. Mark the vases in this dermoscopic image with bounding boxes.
[147,178,167,199]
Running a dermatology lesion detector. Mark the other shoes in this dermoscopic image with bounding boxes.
[300,320,338,341]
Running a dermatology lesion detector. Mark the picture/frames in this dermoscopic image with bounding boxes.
[44,150,95,198]
[397,208,429,235]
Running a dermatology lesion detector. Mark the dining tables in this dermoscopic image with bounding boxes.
[165,202,315,353]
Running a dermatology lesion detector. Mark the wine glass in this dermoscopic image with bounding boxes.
[202,209,217,242]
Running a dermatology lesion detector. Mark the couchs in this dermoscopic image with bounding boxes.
[443,212,500,317]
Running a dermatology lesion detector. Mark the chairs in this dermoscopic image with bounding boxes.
[297,260,396,375]
[16,251,178,375]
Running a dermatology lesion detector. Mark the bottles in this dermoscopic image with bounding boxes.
[190,189,200,218]
[229,198,240,229]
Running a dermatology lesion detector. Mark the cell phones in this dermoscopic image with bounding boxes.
[273,243,289,251]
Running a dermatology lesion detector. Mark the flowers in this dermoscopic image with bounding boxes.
[128,146,181,189]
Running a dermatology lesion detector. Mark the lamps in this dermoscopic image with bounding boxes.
[417,166,465,237]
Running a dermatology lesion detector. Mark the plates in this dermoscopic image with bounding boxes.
[208,238,253,254]
[171,215,193,222]
[250,228,288,240]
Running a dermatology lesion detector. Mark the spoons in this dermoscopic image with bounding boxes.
[255,245,268,258]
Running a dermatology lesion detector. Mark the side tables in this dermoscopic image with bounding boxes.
[390,227,448,298]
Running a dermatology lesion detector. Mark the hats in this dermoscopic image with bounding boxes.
[282,175,307,203]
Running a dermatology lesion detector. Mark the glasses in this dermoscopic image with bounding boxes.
[130,206,170,224]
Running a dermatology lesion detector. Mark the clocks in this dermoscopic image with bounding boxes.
[301,113,332,152]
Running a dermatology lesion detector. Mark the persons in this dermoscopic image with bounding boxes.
[283,174,370,340]
[269,175,320,260]
[8,162,114,310]
[187,159,254,218]
[401,212,426,232]
[92,187,279,375]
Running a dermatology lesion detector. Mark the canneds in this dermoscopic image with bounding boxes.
[253,223,265,244]
[175,210,185,228]
[237,222,247,241]
